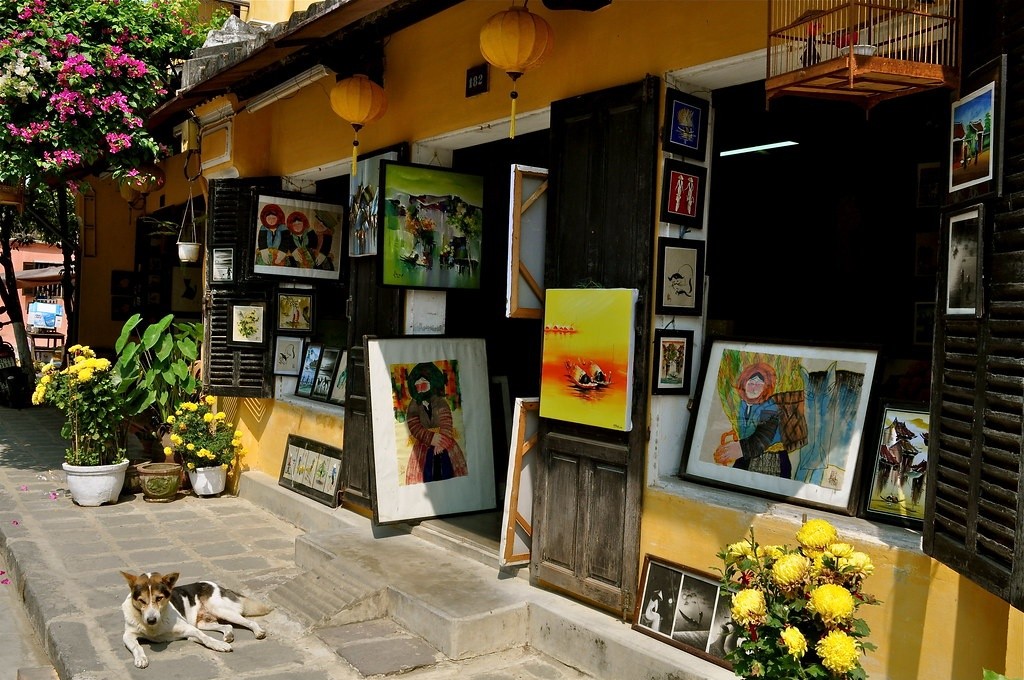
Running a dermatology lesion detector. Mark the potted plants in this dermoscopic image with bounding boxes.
[107,314,204,489]
[139,216,207,262]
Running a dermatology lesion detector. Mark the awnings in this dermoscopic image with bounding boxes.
[0,265,75,288]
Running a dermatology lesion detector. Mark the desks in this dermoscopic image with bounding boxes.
[26,329,65,360]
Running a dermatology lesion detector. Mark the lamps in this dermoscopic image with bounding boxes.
[245,64,331,115]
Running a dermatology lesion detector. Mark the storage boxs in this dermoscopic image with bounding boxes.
[26,303,63,331]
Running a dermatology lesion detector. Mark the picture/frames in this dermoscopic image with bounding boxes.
[272,332,306,378]
[209,243,237,285]
[277,292,312,332]
[248,185,346,286]
[660,158,708,230]
[661,87,711,163]
[940,200,988,320]
[855,394,930,531]
[940,54,1009,210]
[651,328,695,396]
[294,342,324,398]
[654,236,705,316]
[505,163,549,318]
[327,347,348,407]
[310,345,343,403]
[676,332,889,516]
[497,397,540,568]
[379,158,488,293]
[362,333,501,526]
[227,301,269,351]
[278,433,344,509]
[630,552,773,673]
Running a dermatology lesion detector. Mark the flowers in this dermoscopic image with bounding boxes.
[31,344,127,466]
[708,514,884,680]
[164,394,248,477]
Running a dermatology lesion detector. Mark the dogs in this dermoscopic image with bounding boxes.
[120,570,272,669]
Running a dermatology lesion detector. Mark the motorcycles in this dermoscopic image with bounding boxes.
[0,306,19,409]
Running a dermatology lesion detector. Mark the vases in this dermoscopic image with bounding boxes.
[62,458,129,507]
[123,457,153,492]
[186,465,227,499]
[175,241,201,263]
[137,462,184,503]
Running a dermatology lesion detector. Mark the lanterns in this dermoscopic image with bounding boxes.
[329,73,389,178]
[481,7,555,138]
